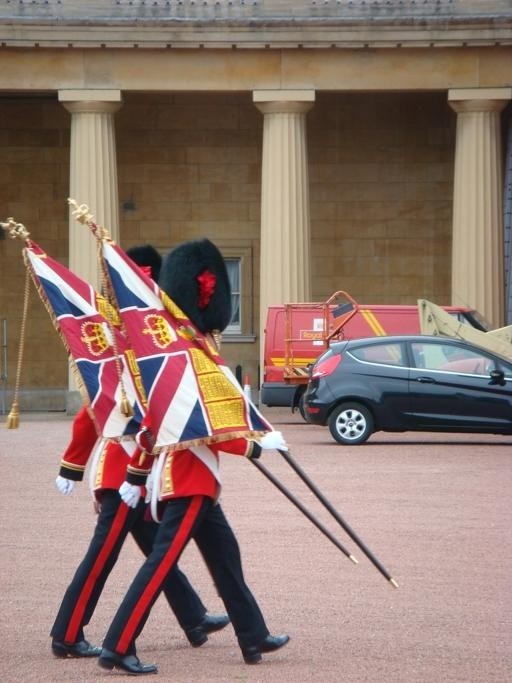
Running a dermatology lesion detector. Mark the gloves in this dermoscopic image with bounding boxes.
[57,476,75,493]
[260,431,288,451]
[118,481,141,508]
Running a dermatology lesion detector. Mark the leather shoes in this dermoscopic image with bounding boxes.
[186,615,230,646]
[100,648,157,675]
[243,635,288,664]
[52,639,102,658]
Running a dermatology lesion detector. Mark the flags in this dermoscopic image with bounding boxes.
[97,237,274,456]
[21,242,139,443]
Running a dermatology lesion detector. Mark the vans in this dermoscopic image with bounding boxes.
[260,290,493,424]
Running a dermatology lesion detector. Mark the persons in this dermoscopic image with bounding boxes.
[50,325,230,659]
[97,330,289,674]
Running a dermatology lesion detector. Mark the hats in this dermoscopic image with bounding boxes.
[158,239,231,333]
[126,245,161,279]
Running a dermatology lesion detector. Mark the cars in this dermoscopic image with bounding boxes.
[299,335,512,445]
